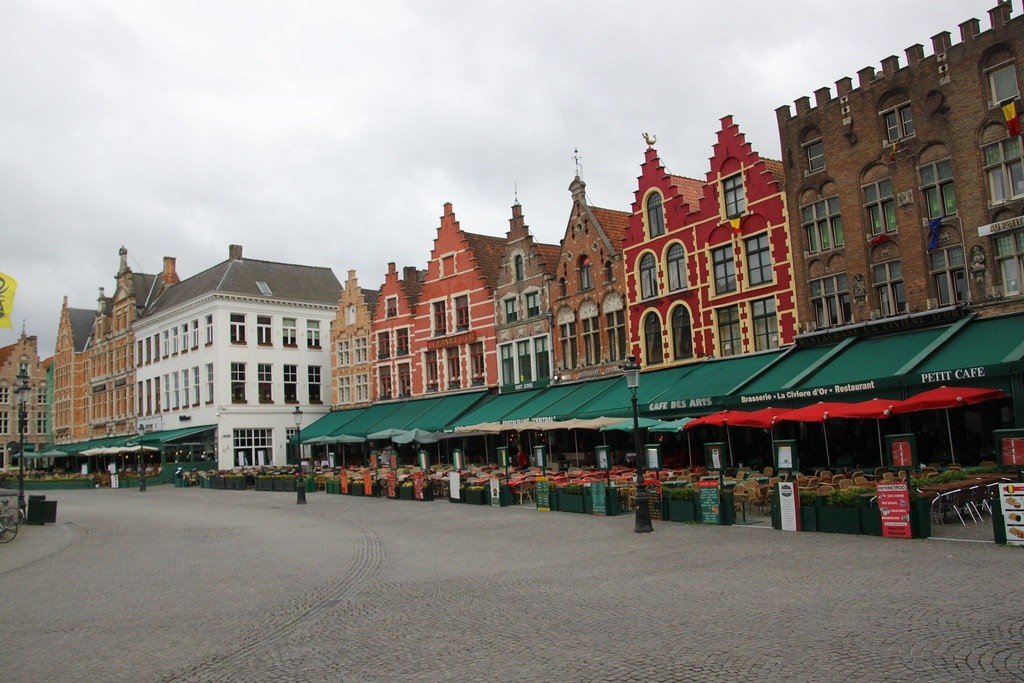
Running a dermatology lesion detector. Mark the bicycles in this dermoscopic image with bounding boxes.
[1,499,25,530]
[1,507,18,544]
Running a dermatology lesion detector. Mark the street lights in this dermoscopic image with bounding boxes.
[623,354,654,534]
[14,380,32,522]
[293,406,308,505]
[139,424,146,491]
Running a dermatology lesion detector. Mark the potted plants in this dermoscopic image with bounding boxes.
[352,480,364,495]
[5,473,161,490]
[400,481,414,499]
[448,485,933,540]
[210,474,341,494]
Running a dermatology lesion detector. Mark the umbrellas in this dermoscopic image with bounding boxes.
[12,445,159,468]
[727,407,797,469]
[826,398,900,467]
[302,416,634,468]
[775,401,852,467]
[888,386,1003,465]
[687,410,747,465]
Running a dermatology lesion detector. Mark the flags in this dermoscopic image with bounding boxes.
[0,273,17,329]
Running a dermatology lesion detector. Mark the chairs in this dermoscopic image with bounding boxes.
[428,460,1020,530]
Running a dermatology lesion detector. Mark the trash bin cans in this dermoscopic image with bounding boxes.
[27,494,57,526]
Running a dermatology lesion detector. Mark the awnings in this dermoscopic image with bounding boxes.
[647,418,691,465]
[602,418,669,444]
